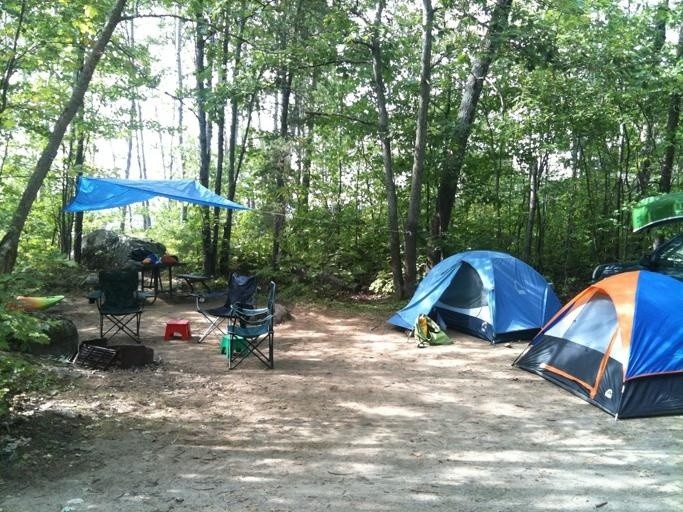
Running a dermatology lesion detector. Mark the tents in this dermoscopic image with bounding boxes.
[387,251,563,345]
[511,270,683,421]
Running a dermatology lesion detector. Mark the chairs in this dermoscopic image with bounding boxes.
[97,269,143,344]
[199,276,277,369]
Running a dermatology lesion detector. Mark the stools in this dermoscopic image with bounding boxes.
[165,319,192,342]
